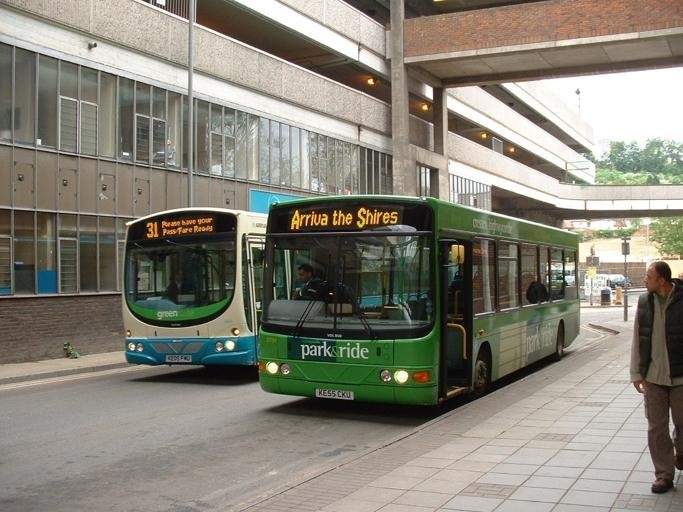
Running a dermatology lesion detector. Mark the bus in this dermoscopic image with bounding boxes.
[256,195,580,407]
[122,206,459,378]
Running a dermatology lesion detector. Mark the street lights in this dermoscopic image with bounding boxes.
[575,87,584,116]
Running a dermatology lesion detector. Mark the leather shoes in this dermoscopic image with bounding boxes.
[673,433,682,472]
[651,480,673,492]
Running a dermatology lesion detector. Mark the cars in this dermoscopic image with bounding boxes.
[585,274,632,290]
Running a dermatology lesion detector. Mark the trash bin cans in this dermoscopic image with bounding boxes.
[601,288,611,305]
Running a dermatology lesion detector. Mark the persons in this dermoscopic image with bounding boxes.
[277,263,323,301]
[677,273,683,281]
[162,270,196,305]
[629,260,683,494]
